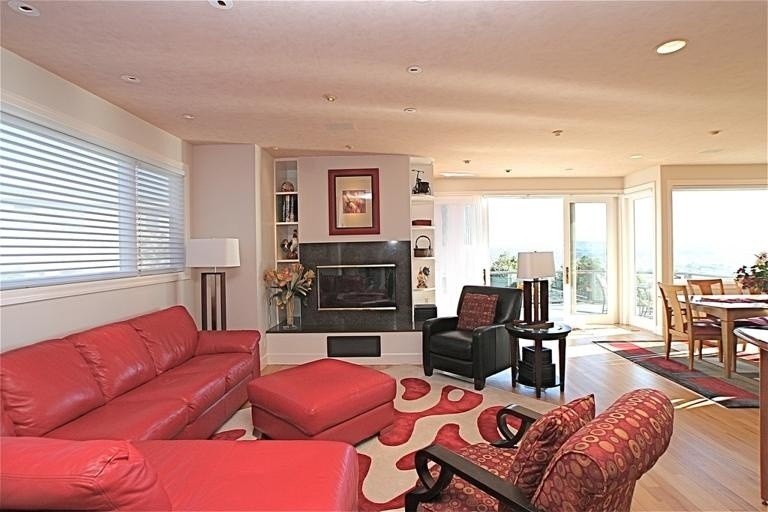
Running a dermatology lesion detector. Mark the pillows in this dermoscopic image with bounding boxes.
[496,393,595,512]
[456,292,499,331]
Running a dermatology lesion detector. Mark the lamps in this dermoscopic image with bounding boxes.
[184,238,240,330]
[517,251,555,327]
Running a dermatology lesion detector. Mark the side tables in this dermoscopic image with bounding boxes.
[505,320,572,399]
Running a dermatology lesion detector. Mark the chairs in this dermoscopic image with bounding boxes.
[657,278,768,371]
[405,388,675,512]
[420,285,525,389]
[594,274,653,320]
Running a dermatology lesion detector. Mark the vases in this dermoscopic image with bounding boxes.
[286,293,295,329]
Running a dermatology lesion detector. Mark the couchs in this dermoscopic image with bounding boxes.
[0,305,261,439]
[0,436,358,512]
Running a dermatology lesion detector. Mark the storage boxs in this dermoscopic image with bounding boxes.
[517,347,555,388]
[414,304,437,332]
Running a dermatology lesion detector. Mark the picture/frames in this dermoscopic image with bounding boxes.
[328,168,380,235]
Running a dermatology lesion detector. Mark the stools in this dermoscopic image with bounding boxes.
[247,358,396,447]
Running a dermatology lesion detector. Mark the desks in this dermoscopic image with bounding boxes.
[733,325,768,507]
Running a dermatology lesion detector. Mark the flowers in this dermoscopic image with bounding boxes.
[734,251,768,290]
[264,263,316,310]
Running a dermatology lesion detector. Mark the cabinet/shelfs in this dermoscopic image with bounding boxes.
[273,157,301,327]
[407,155,437,330]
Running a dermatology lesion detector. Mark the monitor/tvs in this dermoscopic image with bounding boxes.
[316,264,397,311]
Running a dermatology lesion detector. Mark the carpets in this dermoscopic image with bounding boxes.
[592,338,761,409]
[211,363,562,512]
